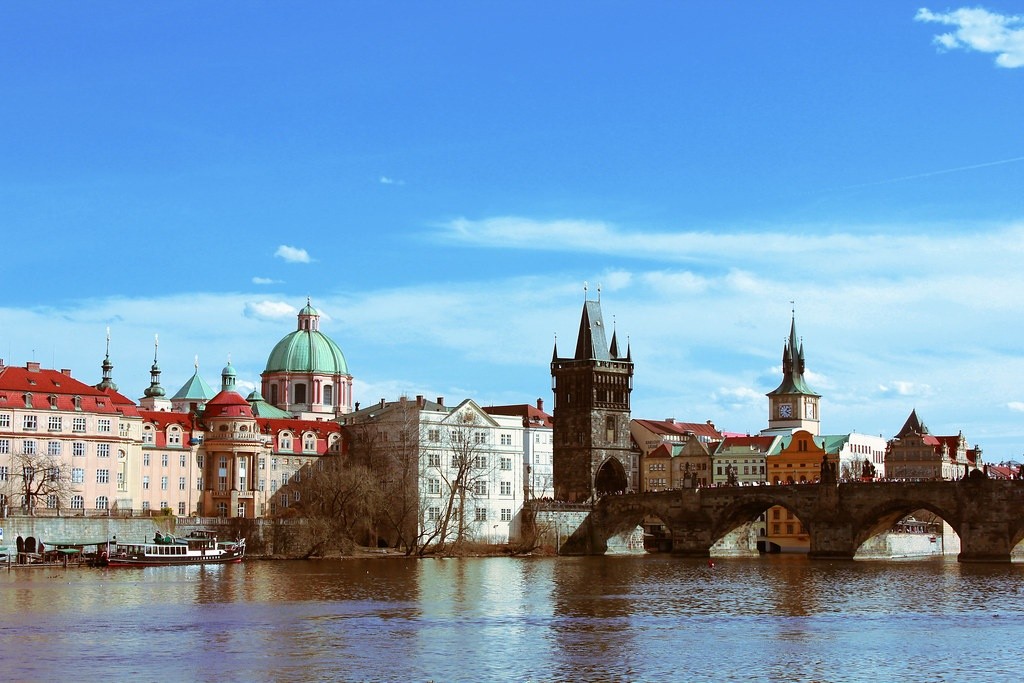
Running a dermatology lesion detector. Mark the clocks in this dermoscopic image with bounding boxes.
[780,405,791,418]
[806,403,813,418]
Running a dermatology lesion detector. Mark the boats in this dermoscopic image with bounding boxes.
[9,540,117,567]
[106,530,246,568]
